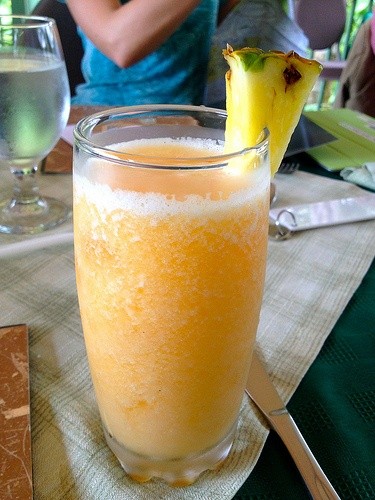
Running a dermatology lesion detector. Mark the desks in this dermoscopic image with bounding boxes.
[0,153,375,500]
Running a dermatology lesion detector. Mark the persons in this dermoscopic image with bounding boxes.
[19,0,375,134]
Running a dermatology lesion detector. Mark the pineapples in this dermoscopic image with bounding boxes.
[220,45,323,179]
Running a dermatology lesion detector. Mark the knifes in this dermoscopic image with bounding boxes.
[245,354,341,500]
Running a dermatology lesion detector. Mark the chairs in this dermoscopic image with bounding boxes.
[295,0,351,113]
[21,0,90,99]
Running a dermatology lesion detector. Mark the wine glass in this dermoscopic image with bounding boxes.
[0,15,71,235]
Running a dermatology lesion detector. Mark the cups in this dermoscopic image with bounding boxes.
[72,104,271,487]
